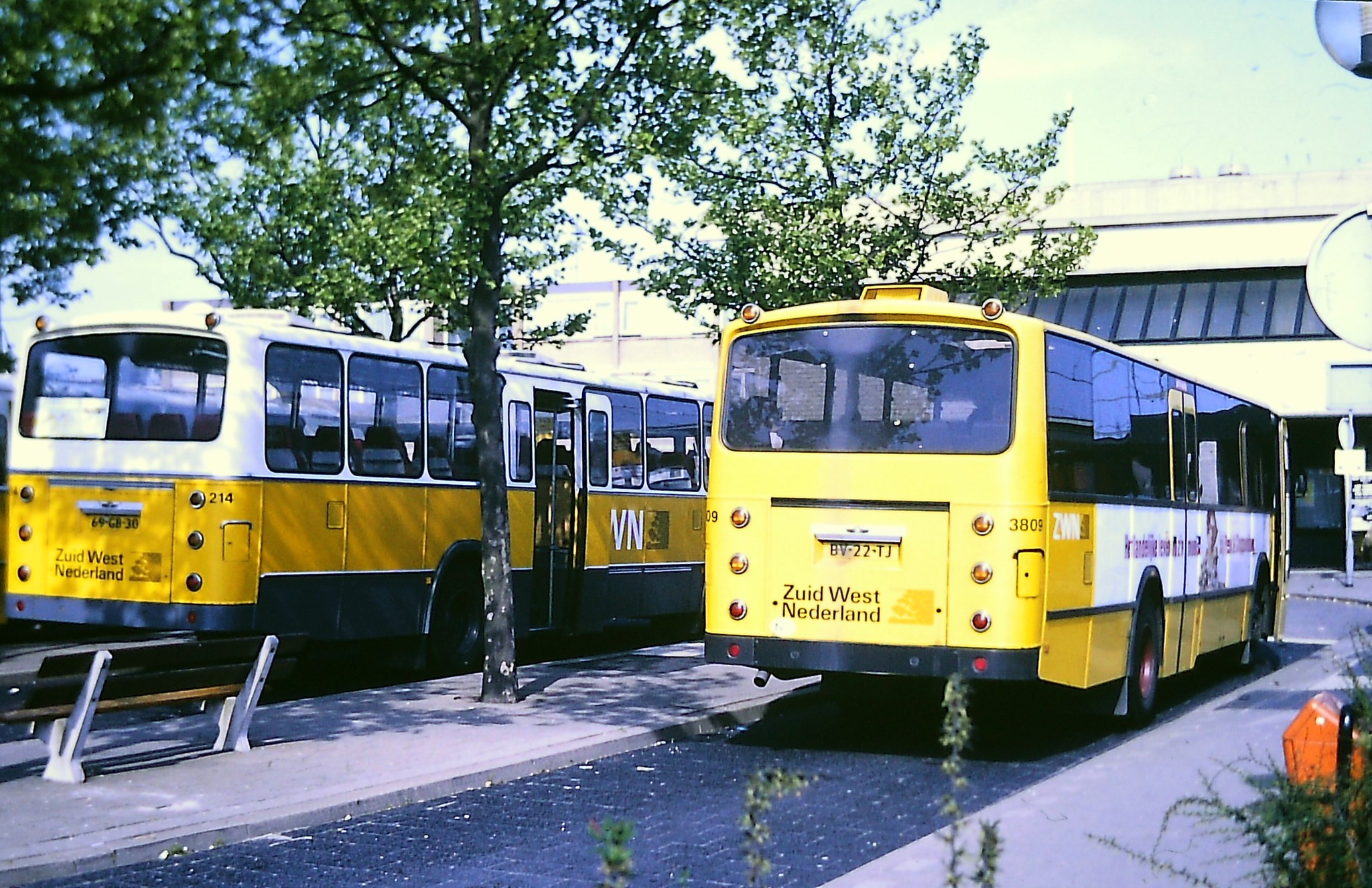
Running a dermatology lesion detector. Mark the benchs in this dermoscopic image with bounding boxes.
[0,630,310,784]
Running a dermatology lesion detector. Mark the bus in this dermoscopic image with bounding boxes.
[1,300,713,677]
[701,283,1307,727]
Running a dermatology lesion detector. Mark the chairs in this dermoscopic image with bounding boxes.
[268,424,306,473]
[648,463,692,489]
[413,433,454,479]
[147,411,186,441]
[612,463,643,486]
[192,413,221,438]
[107,412,144,439]
[361,423,410,477]
[313,426,355,476]
